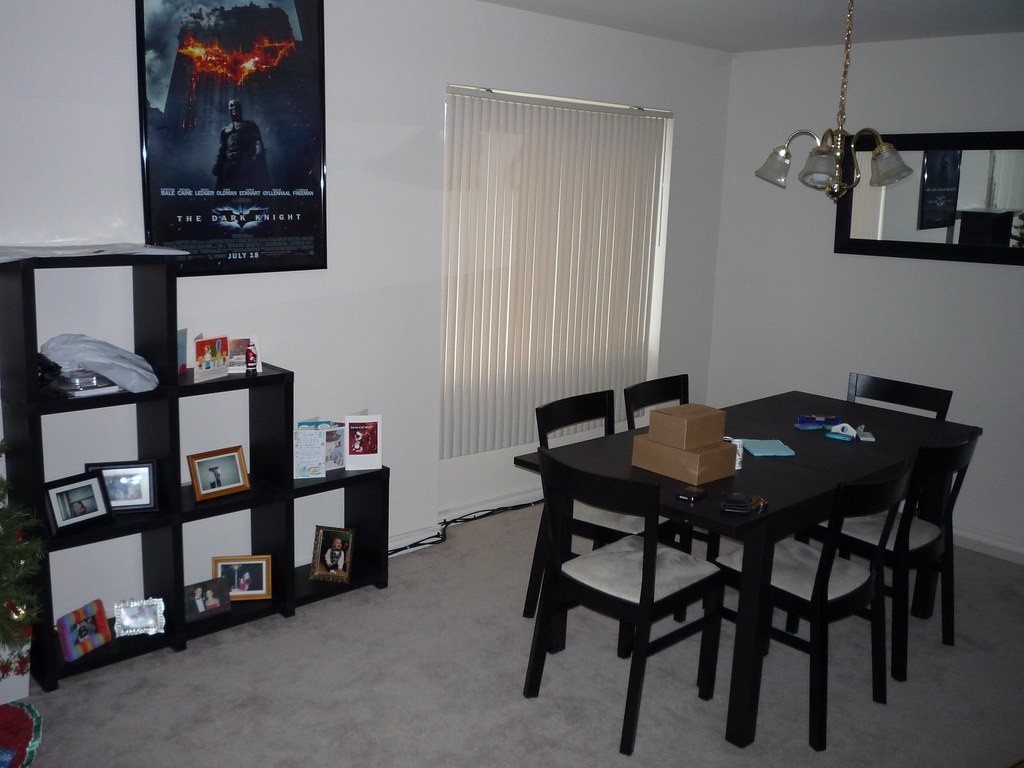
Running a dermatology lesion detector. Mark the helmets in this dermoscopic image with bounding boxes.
[228,96,241,110]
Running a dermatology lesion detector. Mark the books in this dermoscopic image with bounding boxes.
[740,439,795,456]
[68,378,119,397]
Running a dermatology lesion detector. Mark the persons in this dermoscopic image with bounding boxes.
[208,469,221,488]
[78,501,86,514]
[205,589,219,610]
[325,537,347,571]
[190,588,207,613]
[72,620,95,643]
[239,572,250,591]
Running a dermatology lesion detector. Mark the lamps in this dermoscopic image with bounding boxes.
[755,0,915,203]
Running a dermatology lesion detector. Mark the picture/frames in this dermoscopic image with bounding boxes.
[309,525,355,583]
[84,460,158,513]
[918,149,962,231]
[134,0,327,276]
[212,555,272,602]
[186,577,232,623]
[45,468,114,537]
[114,596,165,638]
[57,599,111,663]
[186,446,248,501]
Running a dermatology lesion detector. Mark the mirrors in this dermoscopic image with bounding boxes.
[834,131,1024,267]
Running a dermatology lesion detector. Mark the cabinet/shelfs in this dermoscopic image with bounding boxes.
[0,253,391,694]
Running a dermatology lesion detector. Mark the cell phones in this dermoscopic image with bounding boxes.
[679,486,708,502]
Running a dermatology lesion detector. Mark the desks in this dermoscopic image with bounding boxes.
[513,391,983,748]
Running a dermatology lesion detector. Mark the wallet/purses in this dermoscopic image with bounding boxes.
[720,490,752,514]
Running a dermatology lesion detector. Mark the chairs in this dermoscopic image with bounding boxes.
[521,388,690,659]
[522,445,726,757]
[696,451,921,754]
[624,374,755,600]
[785,427,980,683]
[798,373,954,594]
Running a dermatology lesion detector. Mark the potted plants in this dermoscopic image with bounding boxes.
[0,439,47,707]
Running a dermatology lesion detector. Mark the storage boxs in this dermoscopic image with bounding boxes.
[632,433,737,486]
[648,404,727,451]
